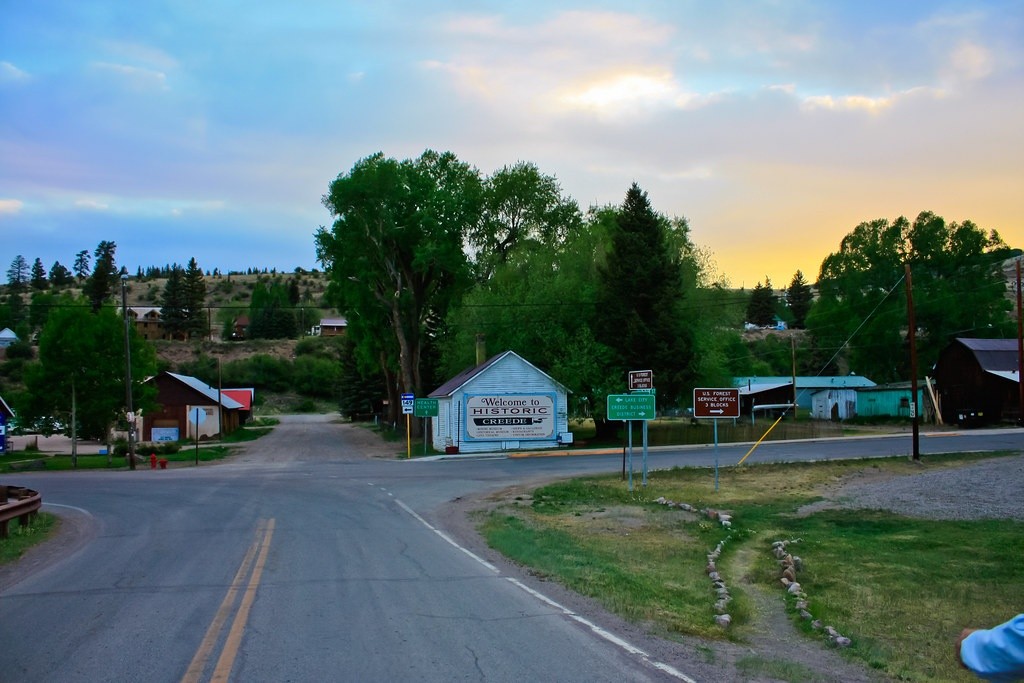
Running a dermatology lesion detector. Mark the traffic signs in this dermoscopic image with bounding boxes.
[693,387,741,418]
[607,394,655,420]
[415,398,439,417]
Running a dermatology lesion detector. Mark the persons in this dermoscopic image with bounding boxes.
[956,612,1023,681]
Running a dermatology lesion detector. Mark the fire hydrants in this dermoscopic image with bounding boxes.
[150,452,168,470]
